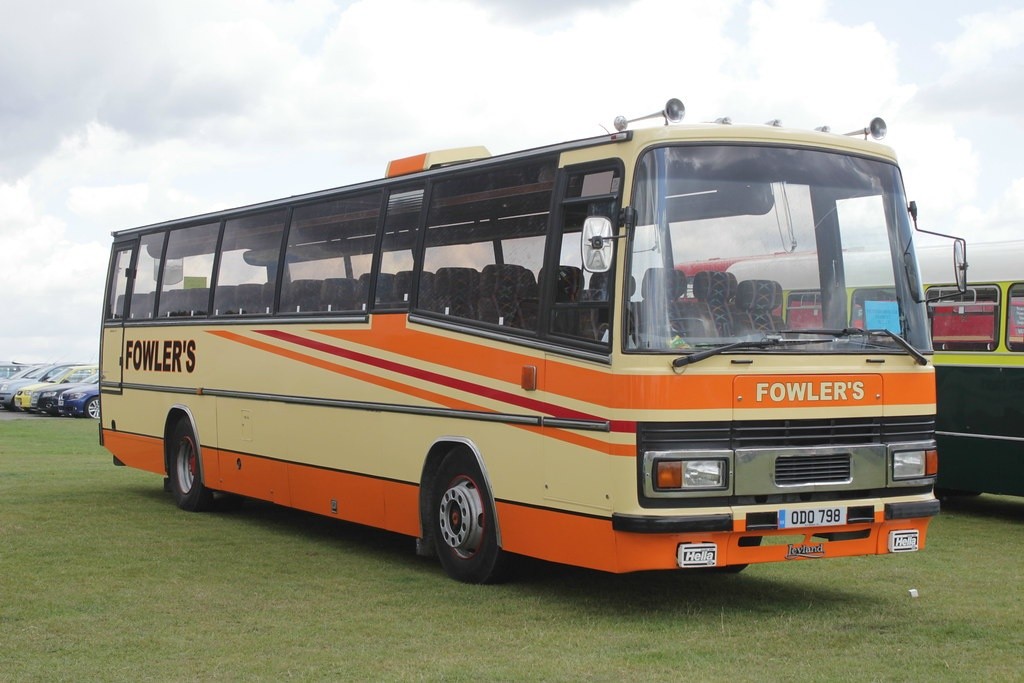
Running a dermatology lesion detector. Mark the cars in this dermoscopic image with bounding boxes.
[0,359,102,420]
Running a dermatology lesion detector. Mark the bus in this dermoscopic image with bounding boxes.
[621,236,1023,503]
[94,99,940,587]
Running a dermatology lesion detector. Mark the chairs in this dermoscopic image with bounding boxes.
[120,264,790,356]
